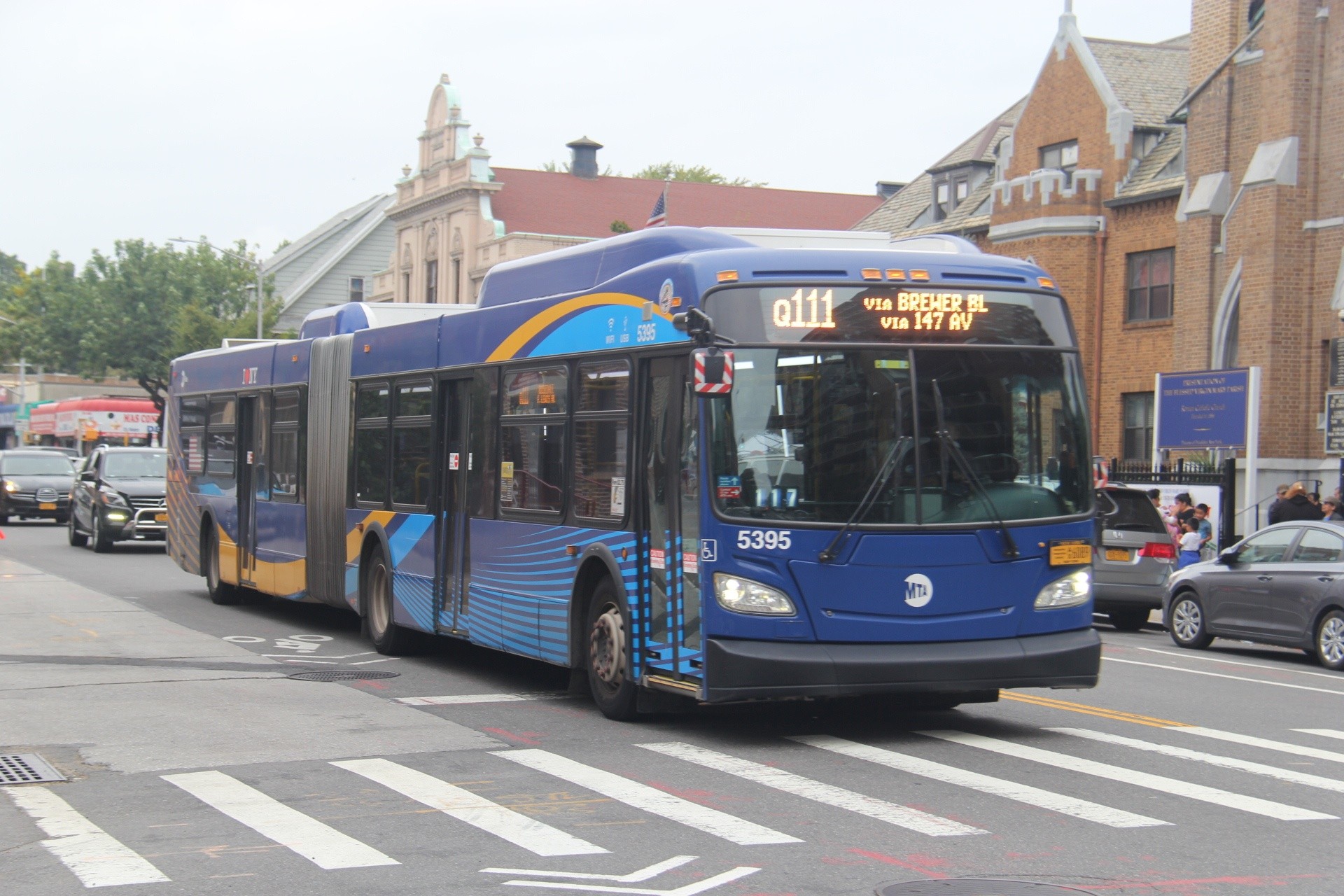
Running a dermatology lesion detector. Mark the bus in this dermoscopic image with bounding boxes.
[166,227,1119,725]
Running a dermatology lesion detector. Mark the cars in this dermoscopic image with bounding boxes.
[0,450,80,526]
[1160,520,1344,672]
[12,446,87,478]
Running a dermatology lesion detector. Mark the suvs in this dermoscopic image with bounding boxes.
[68,442,168,555]
[1014,475,1175,633]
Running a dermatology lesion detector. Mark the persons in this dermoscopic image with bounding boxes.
[1268,483,1344,561]
[592,394,654,490]
[1147,488,1212,568]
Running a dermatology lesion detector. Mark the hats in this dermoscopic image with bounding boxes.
[1319,497,1339,508]
[1276,485,1290,493]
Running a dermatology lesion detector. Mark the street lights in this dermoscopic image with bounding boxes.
[168,238,263,339]
